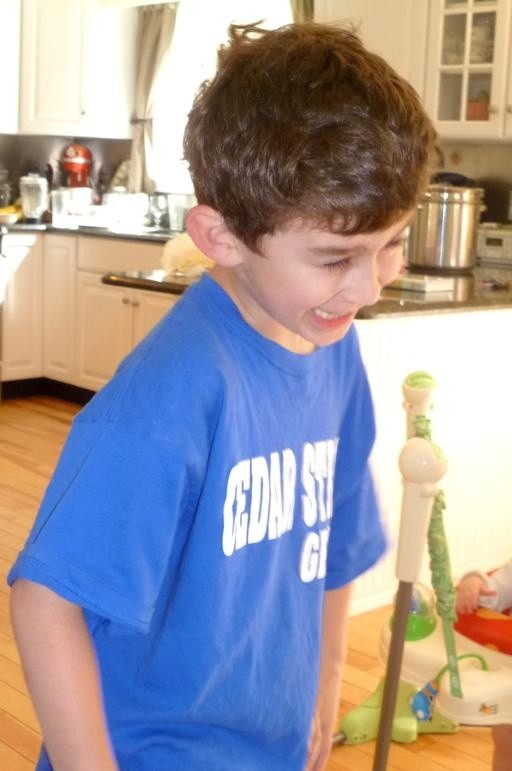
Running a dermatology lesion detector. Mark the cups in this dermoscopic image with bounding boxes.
[19,172,49,224]
[52,190,69,227]
[167,194,185,230]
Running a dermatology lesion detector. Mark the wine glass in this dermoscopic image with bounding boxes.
[149,196,166,229]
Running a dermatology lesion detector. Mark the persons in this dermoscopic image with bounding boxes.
[9,18,443,771]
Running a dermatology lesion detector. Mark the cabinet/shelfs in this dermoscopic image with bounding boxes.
[0,229,182,423]
[1,0,141,144]
[310,0,511,143]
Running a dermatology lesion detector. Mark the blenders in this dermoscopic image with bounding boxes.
[60,143,92,216]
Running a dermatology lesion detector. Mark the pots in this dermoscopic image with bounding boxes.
[406,185,486,270]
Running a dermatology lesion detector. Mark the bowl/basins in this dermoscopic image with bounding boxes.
[463,101,488,119]
[0,216,20,225]
